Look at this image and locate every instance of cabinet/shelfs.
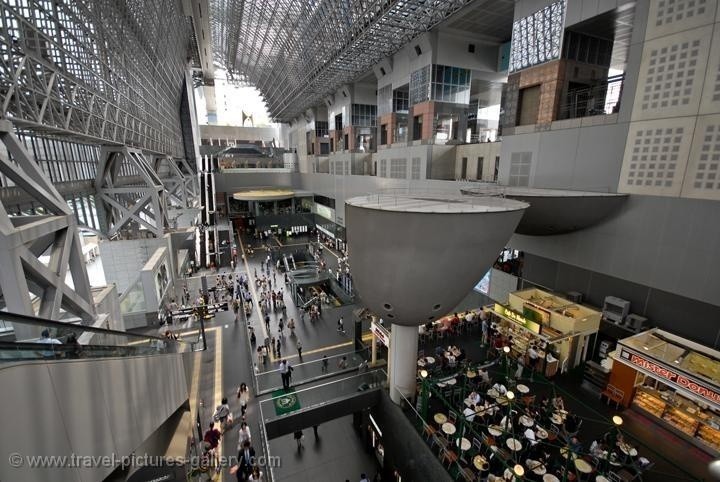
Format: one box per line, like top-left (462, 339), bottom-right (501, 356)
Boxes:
top-left (630, 384), bottom-right (720, 459)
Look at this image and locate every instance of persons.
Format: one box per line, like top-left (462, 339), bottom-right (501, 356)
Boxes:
top-left (156, 221), bottom-right (349, 482)
top-left (217, 206), bottom-right (224, 217)
top-left (417, 307), bottom-right (650, 482)
top-left (64, 331), bottom-right (82, 359)
top-left (359, 467), bottom-right (402, 482)
top-left (35, 329), bottom-right (63, 360)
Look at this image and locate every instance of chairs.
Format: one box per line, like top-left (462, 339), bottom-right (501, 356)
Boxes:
top-left (415, 315), bottom-right (637, 481)
